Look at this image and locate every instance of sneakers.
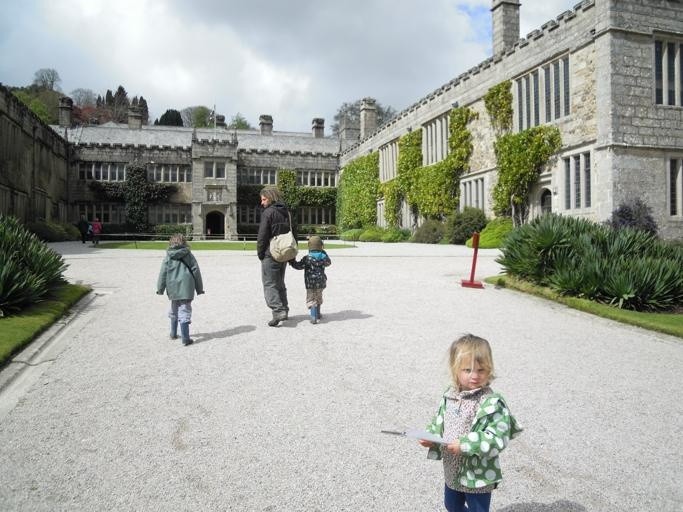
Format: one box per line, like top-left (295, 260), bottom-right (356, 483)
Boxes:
top-left (268, 311), bottom-right (288, 326)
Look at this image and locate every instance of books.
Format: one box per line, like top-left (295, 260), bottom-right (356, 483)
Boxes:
top-left (414, 430), bottom-right (454, 445)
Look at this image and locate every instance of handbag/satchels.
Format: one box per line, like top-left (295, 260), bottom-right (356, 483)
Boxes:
top-left (269, 231), bottom-right (298, 262)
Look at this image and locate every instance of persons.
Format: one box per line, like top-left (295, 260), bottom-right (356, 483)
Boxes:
top-left (155, 234), bottom-right (205, 346)
top-left (91, 217), bottom-right (103, 245)
top-left (256, 185), bottom-right (299, 327)
top-left (77, 215), bottom-right (88, 244)
top-left (288, 235), bottom-right (332, 324)
top-left (414, 333), bottom-right (524, 512)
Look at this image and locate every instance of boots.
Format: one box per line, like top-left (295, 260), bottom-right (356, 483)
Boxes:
top-left (310, 304), bottom-right (320, 324)
top-left (180, 323), bottom-right (193, 345)
top-left (170, 319), bottom-right (177, 338)
